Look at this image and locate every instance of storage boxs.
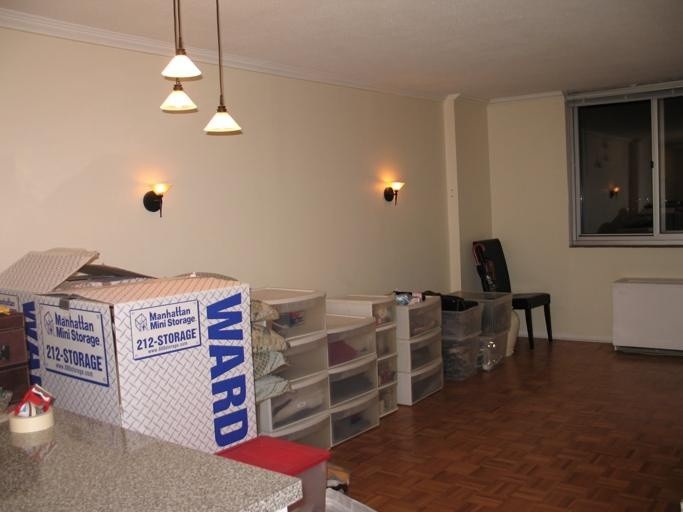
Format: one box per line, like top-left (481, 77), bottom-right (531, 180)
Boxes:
top-left (450, 291), bottom-right (512, 333)
top-left (326, 316), bottom-right (377, 369)
top-left (247, 332), bottom-right (329, 384)
top-left (397, 359), bottom-right (443, 406)
top-left (478, 337), bottom-right (508, 372)
top-left (374, 353), bottom-right (398, 387)
top-left (441, 298), bottom-right (483, 337)
top-left (396, 328), bottom-right (442, 372)
top-left (319, 295), bottom-right (396, 327)
top-left (257, 372), bottom-right (330, 432)
top-left (1, 249), bottom-right (161, 387)
top-left (34, 271), bottom-right (257, 456)
top-left (246, 286), bottom-right (327, 341)
top-left (377, 381), bottom-right (399, 418)
top-left (375, 324), bottom-right (397, 357)
top-left (216, 435), bottom-right (334, 511)
top-left (331, 390), bottom-right (380, 448)
top-left (443, 333), bottom-right (482, 381)
top-left (273, 412), bottom-right (332, 453)
top-left (324, 355), bottom-right (378, 408)
top-left (396, 295), bottom-right (442, 339)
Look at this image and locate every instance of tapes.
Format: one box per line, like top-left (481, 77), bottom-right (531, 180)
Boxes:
top-left (8, 407), bottom-right (56, 434)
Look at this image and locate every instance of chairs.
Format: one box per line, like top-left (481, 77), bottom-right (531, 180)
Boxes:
top-left (471, 238), bottom-right (553, 350)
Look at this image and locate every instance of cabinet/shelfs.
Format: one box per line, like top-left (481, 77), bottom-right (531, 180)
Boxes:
top-left (0, 312), bottom-right (31, 407)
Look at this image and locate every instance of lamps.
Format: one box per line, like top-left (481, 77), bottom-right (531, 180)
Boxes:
top-left (384, 181), bottom-right (404, 207)
top-left (160, 1), bottom-right (197, 111)
top-left (204, 0), bottom-right (241, 132)
top-left (143, 182), bottom-right (172, 218)
top-left (161, 0), bottom-right (202, 79)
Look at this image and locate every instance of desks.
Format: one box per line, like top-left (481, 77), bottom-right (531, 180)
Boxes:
top-left (0, 408), bottom-right (302, 511)
